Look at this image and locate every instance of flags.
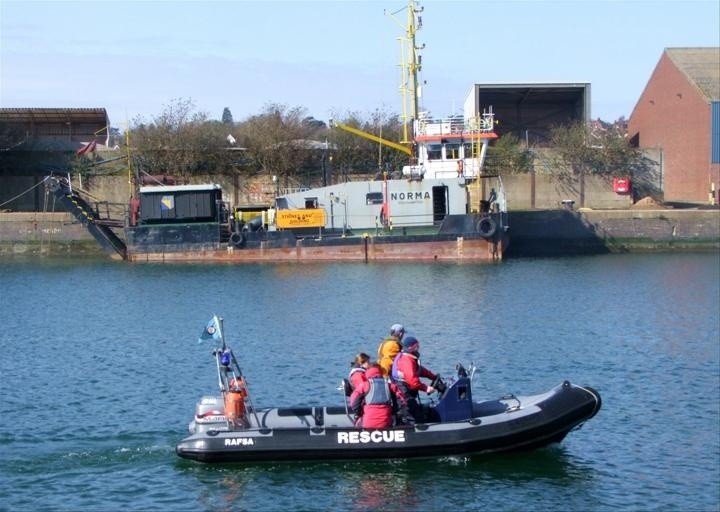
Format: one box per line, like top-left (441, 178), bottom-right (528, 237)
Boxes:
top-left (77, 140), bottom-right (96, 159)
top-left (195, 317), bottom-right (221, 345)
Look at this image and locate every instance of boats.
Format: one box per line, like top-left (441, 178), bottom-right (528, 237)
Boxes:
top-left (43, 0), bottom-right (509, 261)
top-left (175, 313), bottom-right (602, 463)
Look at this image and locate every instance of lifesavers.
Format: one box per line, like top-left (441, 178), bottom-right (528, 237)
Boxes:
top-left (225, 378), bottom-right (246, 426)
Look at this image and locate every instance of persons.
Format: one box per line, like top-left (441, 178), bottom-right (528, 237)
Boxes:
top-left (391, 336), bottom-right (438, 426)
top-left (349, 354), bottom-right (372, 412)
top-left (348, 363), bottom-right (407, 431)
top-left (375, 323), bottom-right (407, 425)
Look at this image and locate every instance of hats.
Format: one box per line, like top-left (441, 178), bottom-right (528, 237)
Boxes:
top-left (403, 337), bottom-right (418, 347)
top-left (390, 324), bottom-right (404, 336)
top-left (365, 367), bottom-right (382, 378)
